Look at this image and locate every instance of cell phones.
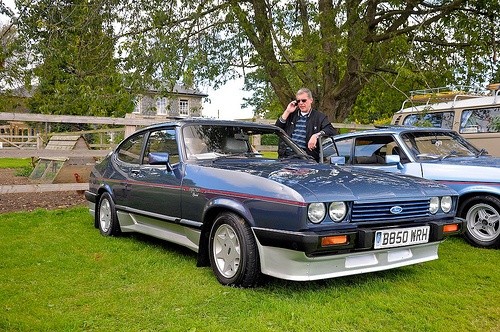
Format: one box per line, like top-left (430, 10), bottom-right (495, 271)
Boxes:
top-left (295, 102), bottom-right (298, 106)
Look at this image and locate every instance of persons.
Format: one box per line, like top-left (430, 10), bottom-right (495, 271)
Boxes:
top-left (275, 88), bottom-right (336, 163)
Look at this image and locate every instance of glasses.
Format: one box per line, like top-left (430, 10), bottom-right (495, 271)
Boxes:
top-left (297, 97), bottom-right (311, 103)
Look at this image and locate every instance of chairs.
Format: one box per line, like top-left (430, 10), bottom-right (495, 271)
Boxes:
top-left (357, 155), bottom-right (384, 164)
top-left (222, 138), bottom-right (248, 156)
top-left (392, 145), bottom-right (411, 164)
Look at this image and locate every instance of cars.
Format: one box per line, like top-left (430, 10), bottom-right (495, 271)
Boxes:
top-left (324, 124), bottom-right (500, 249)
top-left (83, 118), bottom-right (468, 289)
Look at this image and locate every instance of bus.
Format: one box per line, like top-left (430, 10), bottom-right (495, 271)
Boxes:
top-left (390, 85), bottom-right (500, 159)
